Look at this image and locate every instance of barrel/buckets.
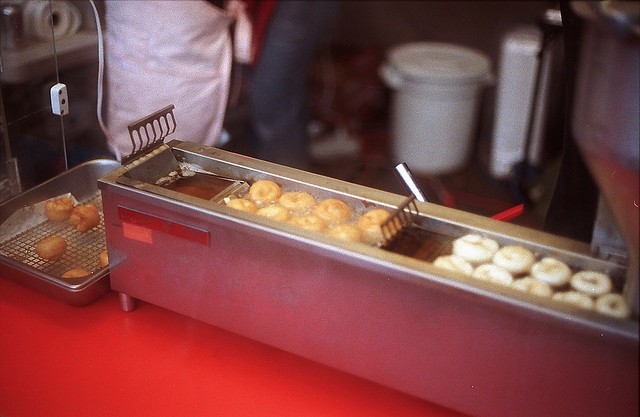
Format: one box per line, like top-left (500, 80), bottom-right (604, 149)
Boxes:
top-left (383, 41), bottom-right (496, 172)
